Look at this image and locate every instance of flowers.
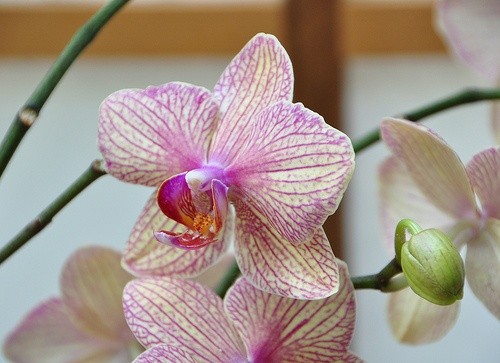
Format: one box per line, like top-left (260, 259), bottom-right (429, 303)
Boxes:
top-left (0, 0), bottom-right (499, 361)
